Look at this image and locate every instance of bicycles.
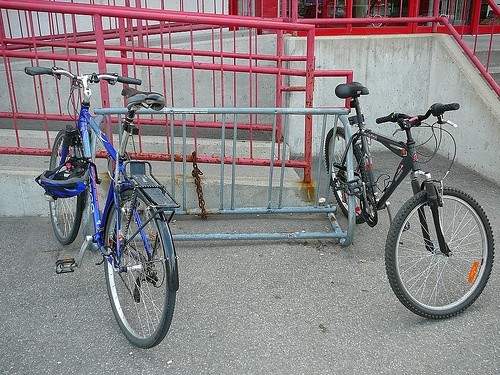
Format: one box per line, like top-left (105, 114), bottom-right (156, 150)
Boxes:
top-left (24, 66), bottom-right (181, 350)
top-left (324, 81), bottom-right (496, 320)
